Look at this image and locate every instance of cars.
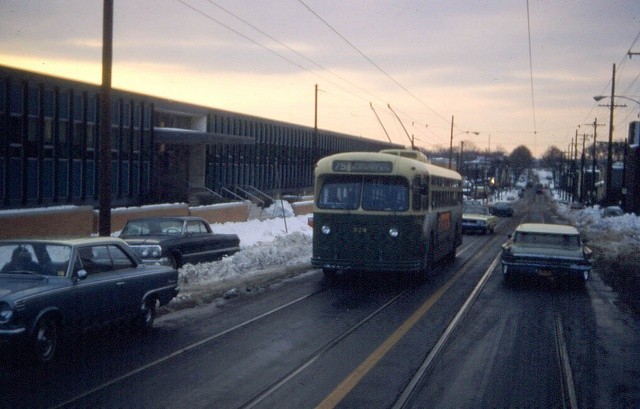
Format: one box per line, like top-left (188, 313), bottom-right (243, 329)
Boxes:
top-left (0, 236), bottom-right (178, 366)
top-left (459, 206), bottom-right (500, 234)
top-left (63, 236), bottom-right (168, 268)
top-left (501, 222), bottom-right (592, 285)
top-left (118, 216), bottom-right (241, 266)
top-left (491, 202), bottom-right (514, 217)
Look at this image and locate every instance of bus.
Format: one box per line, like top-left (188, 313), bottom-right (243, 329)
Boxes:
top-left (310, 149), bottom-right (462, 283)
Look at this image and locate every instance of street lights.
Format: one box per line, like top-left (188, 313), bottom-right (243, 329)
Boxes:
top-left (593, 93), bottom-right (640, 107)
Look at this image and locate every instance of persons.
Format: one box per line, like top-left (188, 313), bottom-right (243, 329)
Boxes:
top-left (327, 187), bottom-right (341, 203)
top-left (0, 247), bottom-right (42, 274)
